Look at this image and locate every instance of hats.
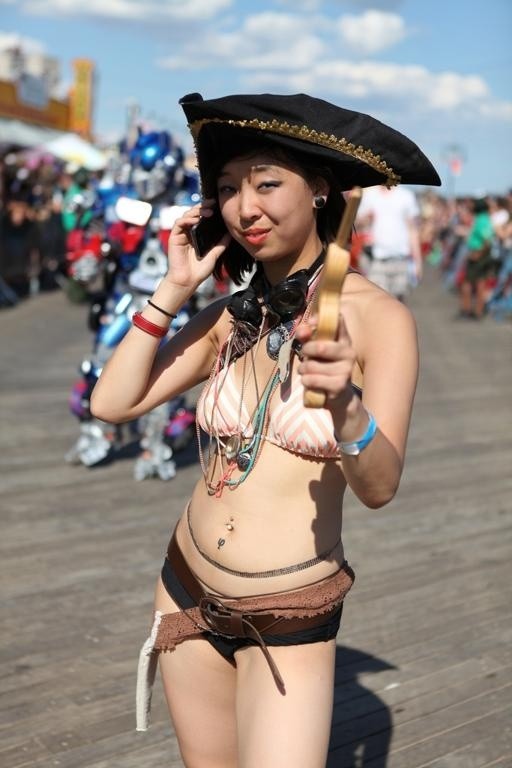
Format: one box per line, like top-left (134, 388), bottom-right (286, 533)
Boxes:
top-left (178, 93), bottom-right (441, 199)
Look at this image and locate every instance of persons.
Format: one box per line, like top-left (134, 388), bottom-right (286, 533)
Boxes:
top-left (84, 145), bottom-right (424, 768)
top-left (1, 146), bottom-right (96, 306)
top-left (61, 126), bottom-right (206, 482)
top-left (352, 181), bottom-right (511, 320)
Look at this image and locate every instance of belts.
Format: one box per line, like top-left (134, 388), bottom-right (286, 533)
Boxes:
top-left (168, 519), bottom-right (342, 696)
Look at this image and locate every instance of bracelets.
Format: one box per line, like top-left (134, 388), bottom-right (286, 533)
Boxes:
top-left (128, 310), bottom-right (170, 340)
top-left (336, 412), bottom-right (380, 457)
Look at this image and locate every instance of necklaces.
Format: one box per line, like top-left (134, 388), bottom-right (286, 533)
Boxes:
top-left (192, 262), bottom-right (327, 500)
